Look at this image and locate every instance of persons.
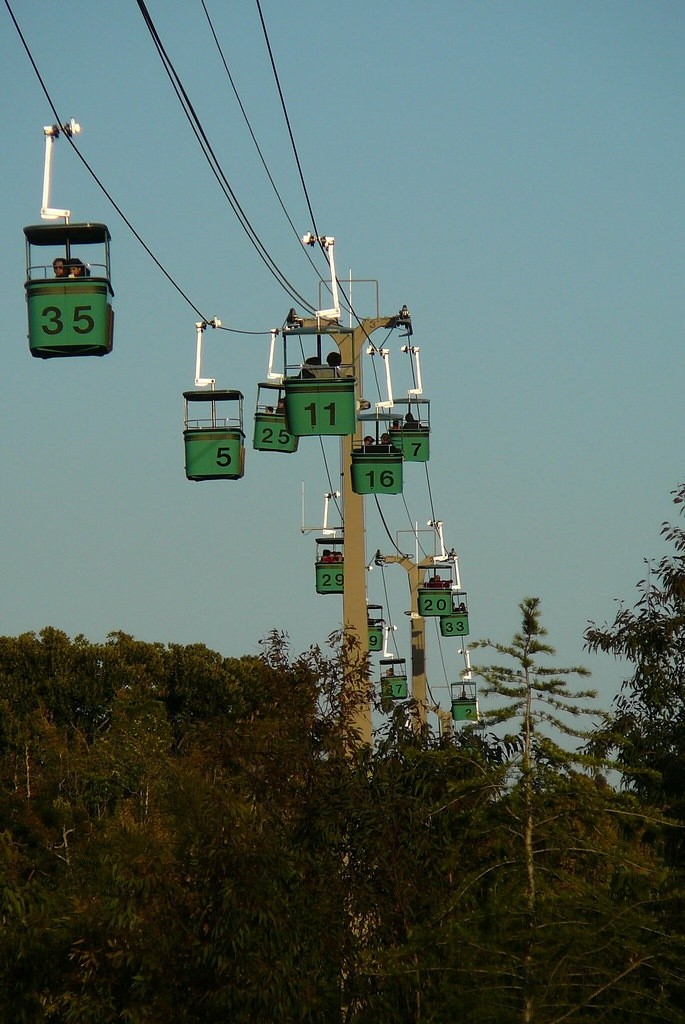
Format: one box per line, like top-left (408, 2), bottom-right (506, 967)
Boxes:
top-left (385, 668), bottom-right (396, 677)
top-left (53, 257), bottom-right (90, 278)
top-left (402, 412), bottom-right (423, 430)
top-left (360, 433), bottom-right (401, 453)
top-left (458, 692), bottom-right (469, 700)
top-left (266, 398), bottom-right (286, 414)
top-left (453, 602), bottom-right (466, 611)
top-left (429, 575), bottom-right (442, 589)
top-left (321, 549), bottom-right (344, 562)
top-left (367, 613), bottom-right (387, 627)
top-left (390, 420), bottom-right (401, 430)
top-left (297, 352), bottom-right (342, 378)
